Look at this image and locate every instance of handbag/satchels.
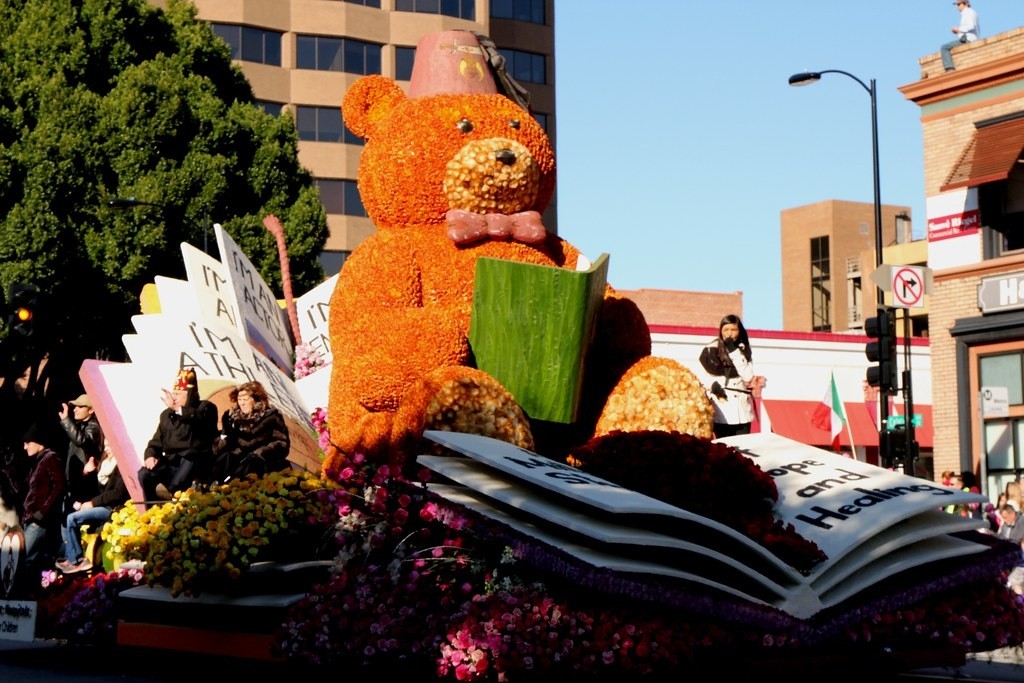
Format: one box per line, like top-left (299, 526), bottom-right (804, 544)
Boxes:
top-left (700, 347), bottom-right (738, 378)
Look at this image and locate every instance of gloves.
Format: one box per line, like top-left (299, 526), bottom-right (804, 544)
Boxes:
top-left (712, 381), bottom-right (727, 399)
top-left (724, 337), bottom-right (737, 352)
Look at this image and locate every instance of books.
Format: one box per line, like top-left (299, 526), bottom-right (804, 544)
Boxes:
top-left (462, 252), bottom-right (610, 426)
top-left (80, 217), bottom-right (347, 505)
top-left (417, 431), bottom-right (991, 619)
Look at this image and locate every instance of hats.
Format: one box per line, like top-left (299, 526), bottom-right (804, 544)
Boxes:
top-left (68, 394), bottom-right (91, 408)
top-left (172, 367), bottom-right (199, 392)
top-left (21, 423), bottom-right (48, 446)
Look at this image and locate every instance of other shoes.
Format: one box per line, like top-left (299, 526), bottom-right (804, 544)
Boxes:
top-left (62, 556), bottom-right (92, 573)
top-left (55, 559), bottom-right (71, 570)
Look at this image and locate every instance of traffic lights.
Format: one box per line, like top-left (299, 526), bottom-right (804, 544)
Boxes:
top-left (7, 280), bottom-right (37, 350)
top-left (864, 312), bottom-right (902, 396)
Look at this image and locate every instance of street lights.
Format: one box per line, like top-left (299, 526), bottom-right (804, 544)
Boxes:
top-left (787, 69), bottom-right (890, 468)
top-left (102, 195), bottom-right (210, 257)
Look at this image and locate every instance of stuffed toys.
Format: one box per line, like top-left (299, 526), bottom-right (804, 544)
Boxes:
top-left (330, 61), bottom-right (713, 477)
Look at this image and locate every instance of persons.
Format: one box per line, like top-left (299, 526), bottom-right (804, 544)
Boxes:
top-left (57, 394), bottom-right (104, 543)
top-left (210, 381), bottom-right (291, 483)
top-left (937, 470), bottom-right (1024, 549)
top-left (17, 430), bottom-right (64, 583)
top-left (53, 434), bottom-right (132, 575)
top-left (137, 365), bottom-right (219, 509)
top-left (694, 315), bottom-right (755, 440)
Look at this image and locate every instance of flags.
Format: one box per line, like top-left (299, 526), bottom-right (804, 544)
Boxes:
top-left (864, 378), bottom-right (899, 433)
top-left (810, 369), bottom-right (849, 455)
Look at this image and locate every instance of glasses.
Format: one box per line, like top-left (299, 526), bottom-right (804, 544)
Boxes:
top-left (949, 482), bottom-right (962, 486)
